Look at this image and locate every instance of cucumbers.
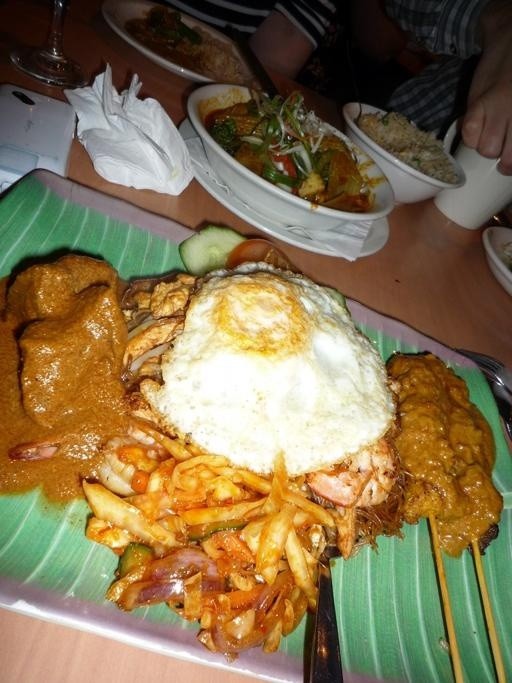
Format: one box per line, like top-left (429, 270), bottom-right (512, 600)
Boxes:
top-left (179, 224), bottom-right (246, 274)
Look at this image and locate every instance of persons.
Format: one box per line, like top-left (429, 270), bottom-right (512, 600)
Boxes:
top-left (153, 0), bottom-right (340, 103)
top-left (379, 0), bottom-right (512, 178)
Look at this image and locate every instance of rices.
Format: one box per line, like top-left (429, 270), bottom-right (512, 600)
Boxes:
top-left (357, 111), bottom-right (456, 184)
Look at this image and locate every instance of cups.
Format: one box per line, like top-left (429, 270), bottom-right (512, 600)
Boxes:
top-left (430, 111), bottom-right (512, 233)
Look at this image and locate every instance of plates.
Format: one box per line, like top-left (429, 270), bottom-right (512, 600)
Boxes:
top-left (0, 164), bottom-right (512, 683)
top-left (177, 116), bottom-right (390, 259)
top-left (101, 0), bottom-right (258, 86)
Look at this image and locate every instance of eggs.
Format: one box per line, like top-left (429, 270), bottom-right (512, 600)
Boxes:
top-left (139, 262), bottom-right (395, 479)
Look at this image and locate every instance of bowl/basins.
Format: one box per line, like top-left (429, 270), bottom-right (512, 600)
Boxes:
top-left (340, 95), bottom-right (467, 205)
top-left (480, 225), bottom-right (512, 297)
top-left (184, 81), bottom-right (397, 231)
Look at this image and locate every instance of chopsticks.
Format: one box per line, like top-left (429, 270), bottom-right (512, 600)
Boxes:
top-left (224, 19), bottom-right (281, 102)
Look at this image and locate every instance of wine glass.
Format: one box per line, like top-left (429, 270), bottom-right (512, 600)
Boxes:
top-left (8, 1), bottom-right (95, 90)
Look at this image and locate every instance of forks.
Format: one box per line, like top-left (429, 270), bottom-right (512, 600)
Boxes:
top-left (451, 344), bottom-right (511, 397)
